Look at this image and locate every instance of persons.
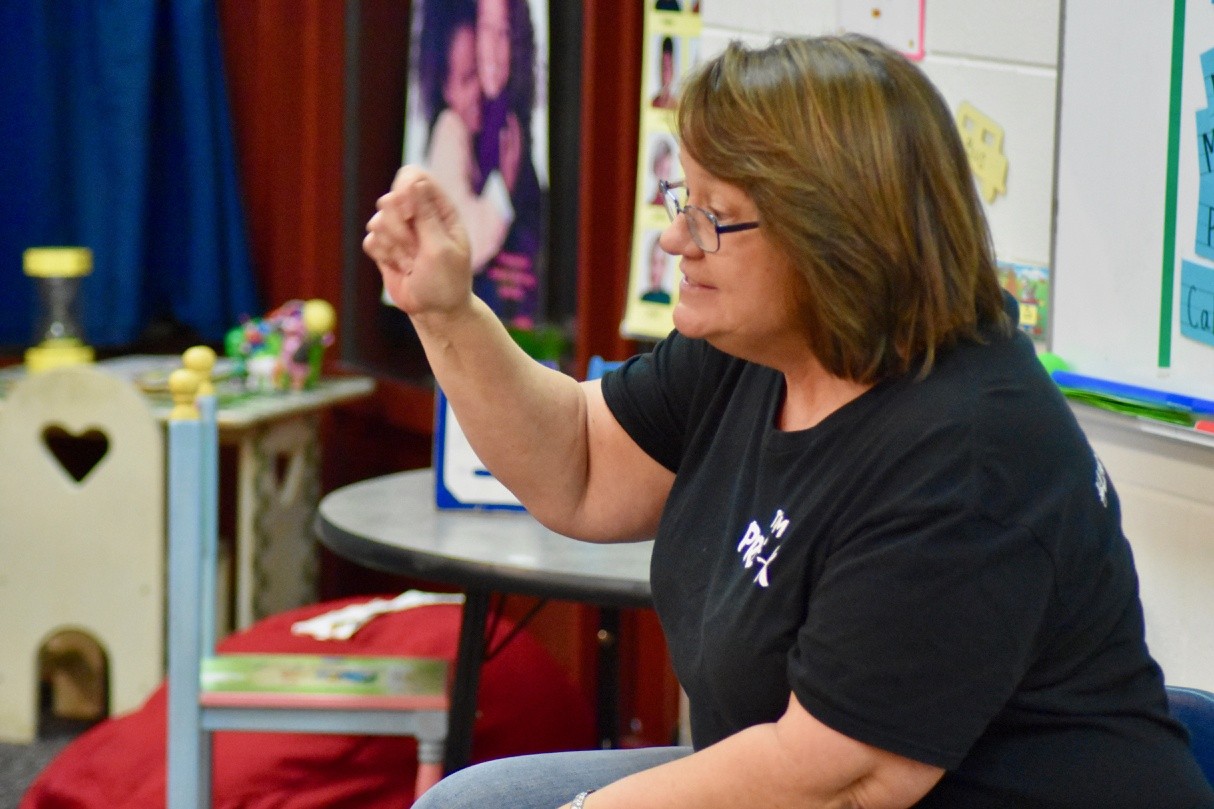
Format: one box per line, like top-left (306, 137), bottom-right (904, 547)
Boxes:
top-left (362, 31), bottom-right (1214, 809)
top-left (419, 0), bottom-right (543, 317)
top-left (650, 141), bottom-right (673, 205)
top-left (641, 237), bottom-right (671, 304)
top-left (653, 37), bottom-right (677, 109)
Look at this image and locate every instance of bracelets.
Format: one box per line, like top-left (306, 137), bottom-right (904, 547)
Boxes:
top-left (570, 789), bottom-right (594, 809)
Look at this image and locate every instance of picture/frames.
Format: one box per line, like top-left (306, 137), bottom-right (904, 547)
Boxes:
top-left (343, 0), bottom-right (584, 390)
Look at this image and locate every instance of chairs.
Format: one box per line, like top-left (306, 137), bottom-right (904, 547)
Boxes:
top-left (166, 347), bottom-right (451, 809)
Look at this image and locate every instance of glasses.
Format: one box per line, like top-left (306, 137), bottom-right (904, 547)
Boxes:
top-left (658, 179), bottom-right (759, 253)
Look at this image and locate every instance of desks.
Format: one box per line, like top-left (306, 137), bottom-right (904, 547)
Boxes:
top-left (306, 465), bottom-right (653, 774)
